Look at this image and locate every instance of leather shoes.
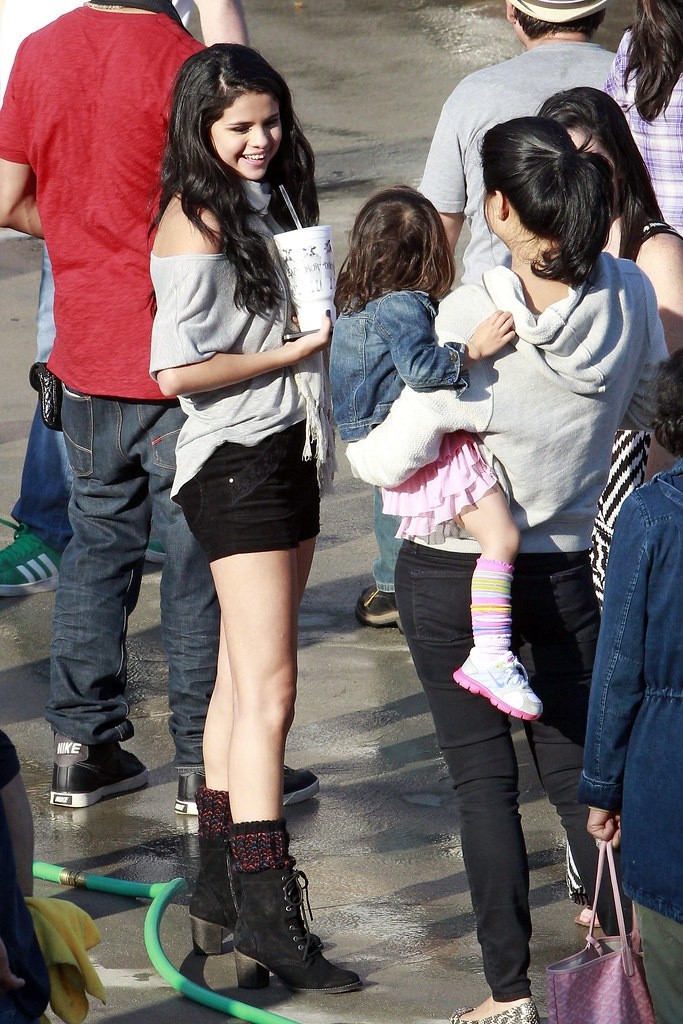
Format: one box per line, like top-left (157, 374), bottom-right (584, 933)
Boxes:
top-left (450, 1000), bottom-right (540, 1024)
top-left (357, 585), bottom-right (403, 633)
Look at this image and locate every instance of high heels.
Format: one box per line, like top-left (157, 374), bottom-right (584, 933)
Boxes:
top-left (233, 865), bottom-right (363, 994)
top-left (190, 836), bottom-right (239, 958)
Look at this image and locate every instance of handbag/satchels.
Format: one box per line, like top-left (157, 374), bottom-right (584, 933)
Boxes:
top-left (546, 840), bottom-right (656, 1024)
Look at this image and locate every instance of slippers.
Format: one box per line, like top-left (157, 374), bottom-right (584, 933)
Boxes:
top-left (574, 905), bottom-right (601, 927)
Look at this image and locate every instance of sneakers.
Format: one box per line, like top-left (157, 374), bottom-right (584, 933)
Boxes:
top-left (174, 765), bottom-right (321, 816)
top-left (48, 733), bottom-right (150, 808)
top-left (453, 651), bottom-right (543, 720)
top-left (0, 519), bottom-right (63, 595)
top-left (145, 538), bottom-right (169, 564)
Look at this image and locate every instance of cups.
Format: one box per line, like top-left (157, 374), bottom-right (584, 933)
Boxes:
top-left (270, 225), bottom-right (336, 333)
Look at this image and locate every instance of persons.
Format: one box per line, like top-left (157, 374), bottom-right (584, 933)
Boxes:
top-left (1, 804), bottom-right (107, 1024)
top-left (326, 186), bottom-right (542, 721)
top-left (577, 346), bottom-right (683, 1024)
top-left (607, 0), bottom-right (683, 236)
top-left (1, 730), bottom-right (32, 896)
top-left (144, 44), bottom-right (360, 994)
top-left (391, 118), bottom-right (667, 1024)
top-left (0, 0), bottom-right (194, 601)
top-left (415, 0), bottom-right (615, 285)
top-left (536, 87), bottom-right (682, 355)
top-left (0, 0), bottom-right (323, 816)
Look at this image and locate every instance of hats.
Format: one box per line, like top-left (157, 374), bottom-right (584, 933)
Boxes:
top-left (509, 0), bottom-right (609, 23)
